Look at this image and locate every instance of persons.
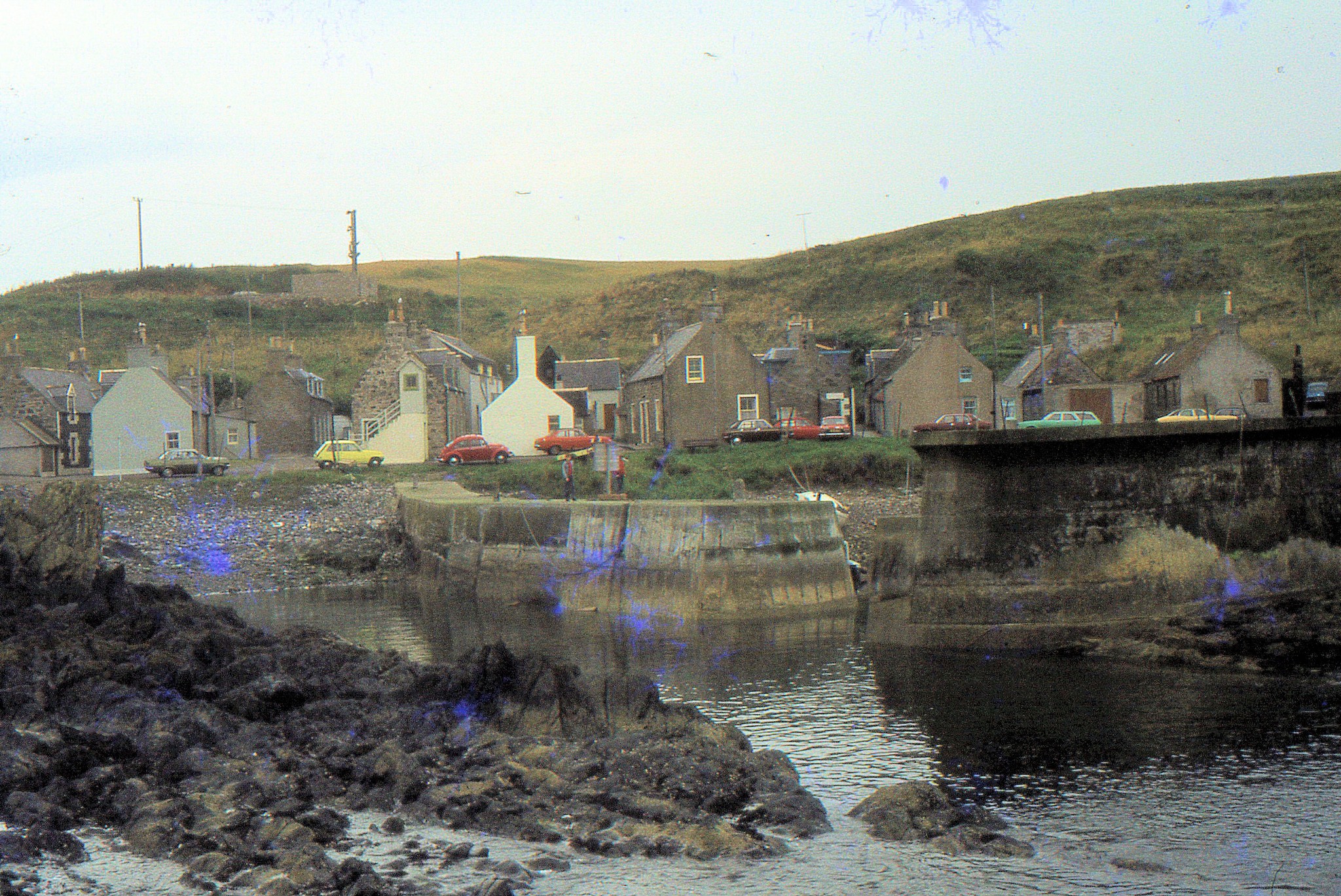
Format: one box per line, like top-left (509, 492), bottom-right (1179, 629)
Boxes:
top-left (614, 455), bottom-right (626, 496)
top-left (562, 454), bottom-right (577, 503)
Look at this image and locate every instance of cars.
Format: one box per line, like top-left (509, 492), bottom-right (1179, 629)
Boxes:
top-left (911, 412), bottom-right (993, 432)
top-left (435, 434), bottom-right (513, 466)
top-left (721, 417), bottom-right (782, 444)
top-left (1016, 411), bottom-right (1104, 429)
top-left (144, 448), bottom-right (233, 482)
top-left (770, 417), bottom-right (822, 441)
top-left (534, 428), bottom-right (615, 456)
top-left (1152, 407), bottom-right (1240, 423)
top-left (1303, 380), bottom-right (1332, 409)
top-left (313, 439), bottom-right (387, 469)
top-left (816, 416), bottom-right (852, 440)
top-left (1216, 407), bottom-right (1246, 418)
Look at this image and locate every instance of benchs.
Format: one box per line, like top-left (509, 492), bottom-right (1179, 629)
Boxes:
top-left (682, 438), bottom-right (719, 453)
